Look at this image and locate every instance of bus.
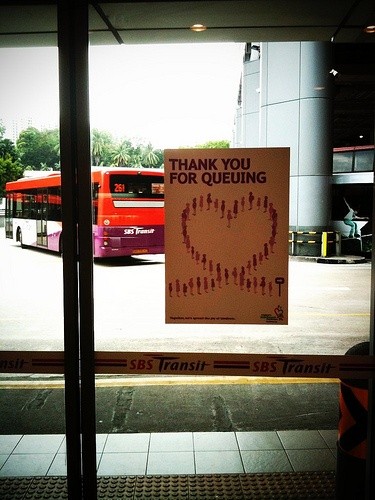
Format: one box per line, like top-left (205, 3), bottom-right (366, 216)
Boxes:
top-left (5, 163), bottom-right (166, 262)
